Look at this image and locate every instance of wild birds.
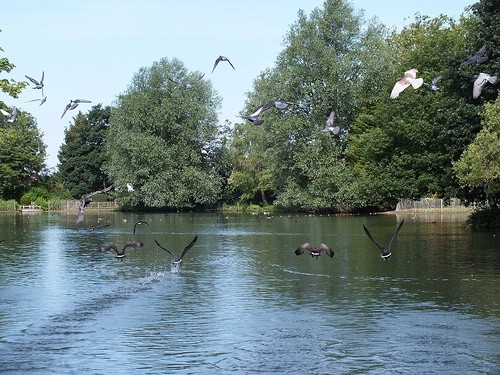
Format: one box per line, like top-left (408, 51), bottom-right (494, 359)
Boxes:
top-left (76, 185), bottom-right (112, 224)
top-left (36, 131), bottom-right (45, 138)
top-left (265, 99), bottom-right (295, 113)
top-left (154, 235), bottom-right (198, 266)
top-left (211, 55), bottom-right (235, 72)
top-left (297, 104), bottom-right (309, 110)
top-left (127, 183), bottom-right (134, 193)
top-left (25, 71), bottom-right (45, 90)
top-left (99, 241), bottom-right (143, 262)
top-left (28, 145), bottom-right (41, 156)
top-left (167, 73), bottom-right (205, 89)
top-left (133, 221), bottom-right (153, 235)
top-left (60, 99), bottom-right (92, 120)
top-left (294, 242), bottom-right (337, 258)
top-left (0, 106), bottom-right (18, 123)
top-left (237, 103), bottom-right (267, 125)
top-left (390, 45), bottom-right (497, 99)
top-left (364, 220), bottom-right (405, 261)
top-left (26, 88), bottom-right (47, 107)
top-left (322, 112), bottom-right (345, 136)
